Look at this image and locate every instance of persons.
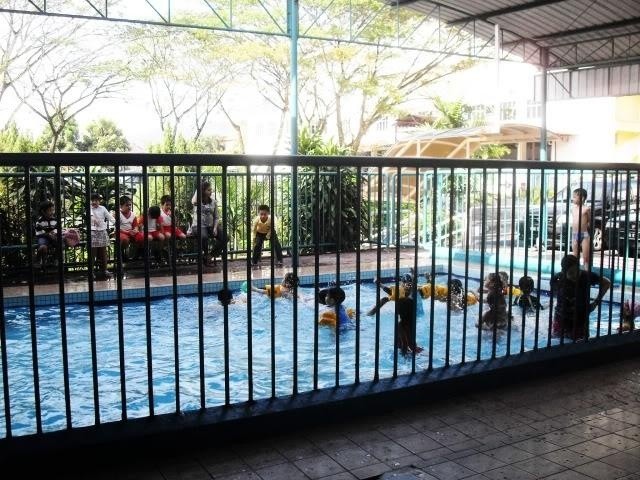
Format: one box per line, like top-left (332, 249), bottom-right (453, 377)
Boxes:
top-left (216, 289), bottom-right (237, 303)
top-left (319, 287), bottom-right (351, 326)
top-left (34, 201), bottom-right (57, 266)
top-left (251, 272), bottom-right (300, 299)
top-left (251, 204), bottom-right (283, 266)
top-left (617, 299), bottom-right (640, 330)
top-left (396, 282), bottom-right (423, 353)
top-left (137, 205), bottom-right (166, 249)
top-left (550, 255), bottom-right (611, 340)
top-left (114, 196), bottom-right (144, 250)
top-left (160, 194), bottom-right (187, 241)
top-left (191, 181), bottom-right (230, 266)
top-left (90, 192), bottom-right (116, 278)
top-left (367, 272), bottom-right (542, 329)
top-left (571, 188), bottom-right (591, 271)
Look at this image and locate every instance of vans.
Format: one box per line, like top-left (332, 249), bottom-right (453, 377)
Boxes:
top-left (515, 180), bottom-right (636, 250)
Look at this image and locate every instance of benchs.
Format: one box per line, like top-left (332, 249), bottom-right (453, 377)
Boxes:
top-left (0, 239), bottom-right (69, 285)
top-left (125, 232), bottom-right (217, 272)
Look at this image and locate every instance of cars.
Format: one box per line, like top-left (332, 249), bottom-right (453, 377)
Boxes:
top-left (605, 207), bottom-right (639, 259)
